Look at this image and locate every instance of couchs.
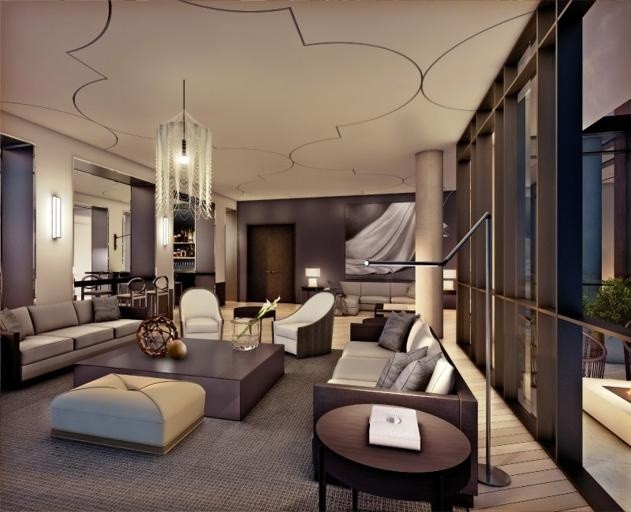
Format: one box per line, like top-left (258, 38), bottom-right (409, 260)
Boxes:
top-left (314, 322), bottom-right (479, 499)
top-left (272, 291), bottom-right (335, 360)
top-left (324, 278), bottom-right (415, 315)
top-left (180, 286), bottom-right (224, 341)
top-left (0, 302), bottom-right (146, 390)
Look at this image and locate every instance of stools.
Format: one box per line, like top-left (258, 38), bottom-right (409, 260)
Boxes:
top-left (49, 373), bottom-right (207, 454)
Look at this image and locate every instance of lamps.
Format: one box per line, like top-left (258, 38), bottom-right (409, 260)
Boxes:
top-left (52, 195), bottom-right (61, 240)
top-left (304, 267), bottom-right (321, 288)
top-left (162, 217), bottom-right (170, 248)
top-left (156, 78), bottom-right (214, 221)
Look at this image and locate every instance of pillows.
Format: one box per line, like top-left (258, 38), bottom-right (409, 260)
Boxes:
top-left (328, 280), bottom-right (345, 295)
top-left (27, 302), bottom-right (79, 335)
top-left (376, 347), bottom-right (428, 391)
top-left (93, 294), bottom-right (120, 319)
top-left (0, 307), bottom-right (23, 342)
top-left (73, 298), bottom-right (93, 324)
top-left (10, 306), bottom-right (34, 336)
top-left (390, 350), bottom-right (443, 392)
top-left (377, 312), bottom-right (417, 352)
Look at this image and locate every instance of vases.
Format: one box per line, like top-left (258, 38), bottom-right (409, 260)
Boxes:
top-left (231, 320), bottom-right (260, 351)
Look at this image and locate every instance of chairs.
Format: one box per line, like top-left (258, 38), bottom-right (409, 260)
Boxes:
top-left (118, 277), bottom-right (148, 314)
top-left (149, 274), bottom-right (170, 317)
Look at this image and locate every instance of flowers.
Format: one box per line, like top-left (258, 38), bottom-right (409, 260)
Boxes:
top-left (237, 296), bottom-right (280, 339)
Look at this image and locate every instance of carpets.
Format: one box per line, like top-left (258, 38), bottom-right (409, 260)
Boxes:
top-left (1, 349), bottom-right (470, 512)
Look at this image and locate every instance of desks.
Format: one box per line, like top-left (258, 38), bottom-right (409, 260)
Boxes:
top-left (74, 276), bottom-right (156, 305)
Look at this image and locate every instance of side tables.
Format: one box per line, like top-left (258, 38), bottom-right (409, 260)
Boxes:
top-left (233, 306), bottom-right (276, 345)
top-left (314, 404), bottom-right (471, 512)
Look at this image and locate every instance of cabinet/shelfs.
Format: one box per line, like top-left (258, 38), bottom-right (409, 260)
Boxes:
top-left (174, 204), bottom-right (214, 273)
